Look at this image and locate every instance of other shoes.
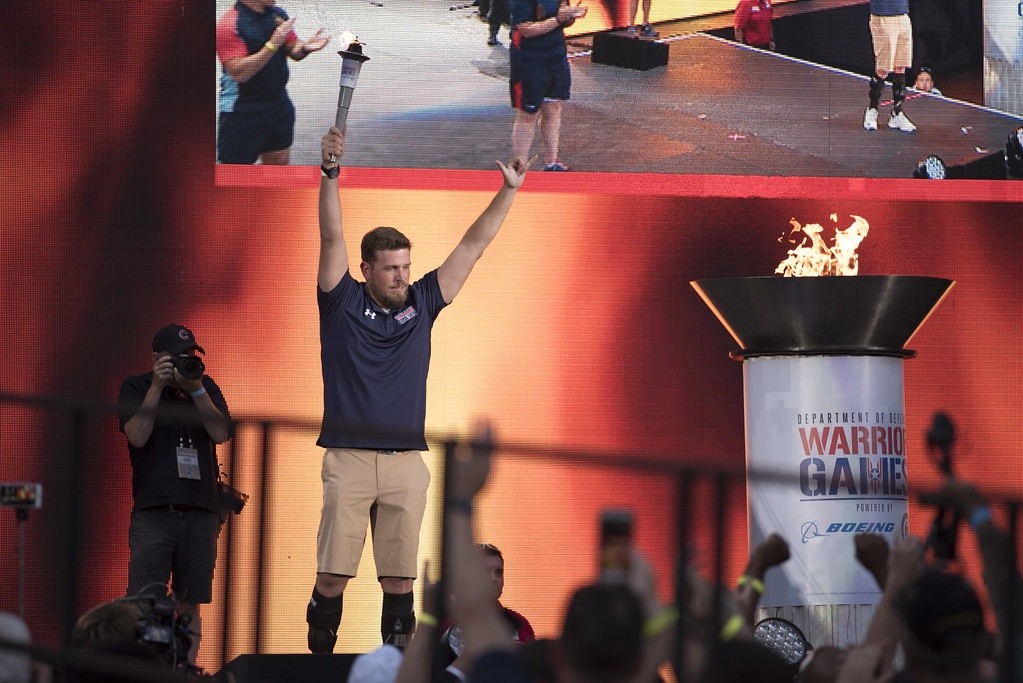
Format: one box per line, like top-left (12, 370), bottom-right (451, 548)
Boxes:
top-left (641, 25), bottom-right (658, 37)
top-left (487, 37), bottom-right (496, 45)
top-left (544, 163), bottom-right (571, 172)
top-left (628, 28), bottom-right (639, 39)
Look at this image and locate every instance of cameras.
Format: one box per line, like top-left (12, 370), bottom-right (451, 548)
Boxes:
top-left (167, 352), bottom-right (205, 380)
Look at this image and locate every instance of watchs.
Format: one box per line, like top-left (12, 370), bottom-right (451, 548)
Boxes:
top-left (321, 164), bottom-right (340, 179)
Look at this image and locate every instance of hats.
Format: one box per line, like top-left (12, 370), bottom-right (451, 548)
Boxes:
top-left (153, 324), bottom-right (206, 356)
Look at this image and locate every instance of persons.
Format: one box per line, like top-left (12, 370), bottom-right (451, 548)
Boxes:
top-left (304, 125), bottom-right (537, 653)
top-left (476, 0), bottom-right (588, 172)
top-left (733, 0), bottom-right (776, 52)
top-left (116, 322), bottom-right (233, 664)
top-left (628, 0), bottom-right (658, 39)
top-left (863, 0), bottom-right (917, 131)
top-left (216, 0), bottom-right (332, 166)
top-left (912, 67), bottom-right (942, 95)
top-left (0, 414), bottom-right (1023, 683)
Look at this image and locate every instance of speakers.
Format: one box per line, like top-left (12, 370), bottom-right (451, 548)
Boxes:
top-left (210, 654), bottom-right (364, 683)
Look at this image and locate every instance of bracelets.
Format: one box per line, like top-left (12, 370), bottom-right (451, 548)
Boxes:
top-left (445, 498), bottom-right (472, 513)
top-left (419, 612), bottom-right (439, 626)
top-left (190, 386), bottom-right (206, 397)
top-left (969, 507), bottom-right (989, 525)
top-left (556, 16), bottom-right (563, 26)
top-left (719, 613), bottom-right (745, 640)
top-left (641, 605), bottom-right (678, 635)
top-left (265, 41), bottom-right (278, 53)
top-left (738, 574), bottom-right (765, 594)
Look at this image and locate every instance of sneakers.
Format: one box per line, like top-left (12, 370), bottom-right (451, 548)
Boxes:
top-left (888, 111), bottom-right (915, 132)
top-left (864, 106), bottom-right (878, 130)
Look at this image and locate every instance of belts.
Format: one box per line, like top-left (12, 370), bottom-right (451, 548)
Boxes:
top-left (152, 502), bottom-right (202, 512)
